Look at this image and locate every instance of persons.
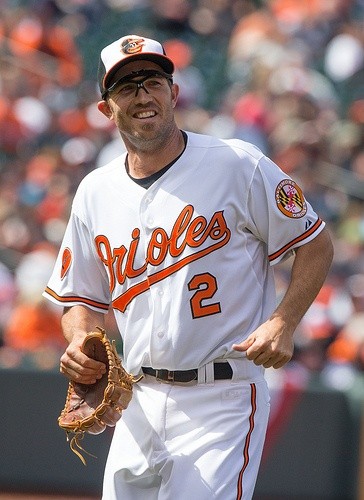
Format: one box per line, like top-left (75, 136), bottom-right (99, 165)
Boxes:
top-left (2, 0), bottom-right (364, 374)
top-left (319, 311), bottom-right (364, 500)
top-left (40, 31), bottom-right (334, 500)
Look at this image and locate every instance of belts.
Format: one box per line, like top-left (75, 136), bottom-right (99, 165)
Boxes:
top-left (138, 360), bottom-right (232, 383)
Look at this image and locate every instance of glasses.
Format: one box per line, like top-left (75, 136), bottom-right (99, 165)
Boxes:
top-left (105, 70), bottom-right (170, 99)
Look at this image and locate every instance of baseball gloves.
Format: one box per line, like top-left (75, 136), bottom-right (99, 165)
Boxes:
top-left (59, 326), bottom-right (145, 465)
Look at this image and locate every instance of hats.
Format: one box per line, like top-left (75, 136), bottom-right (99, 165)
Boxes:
top-left (98, 35), bottom-right (175, 96)
